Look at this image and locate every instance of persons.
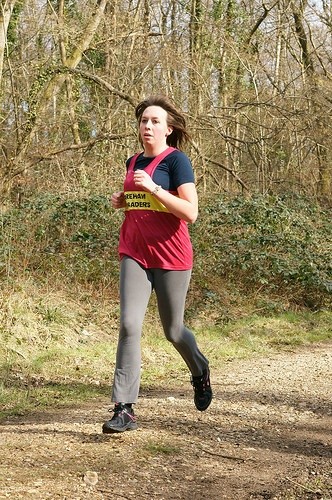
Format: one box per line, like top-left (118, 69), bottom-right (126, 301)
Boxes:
top-left (102, 95), bottom-right (213, 432)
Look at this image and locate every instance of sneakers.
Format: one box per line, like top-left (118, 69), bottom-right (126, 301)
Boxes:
top-left (190, 365), bottom-right (212, 412)
top-left (102, 402), bottom-right (137, 432)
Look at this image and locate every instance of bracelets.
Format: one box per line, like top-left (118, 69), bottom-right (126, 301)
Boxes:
top-left (151, 185), bottom-right (161, 195)
